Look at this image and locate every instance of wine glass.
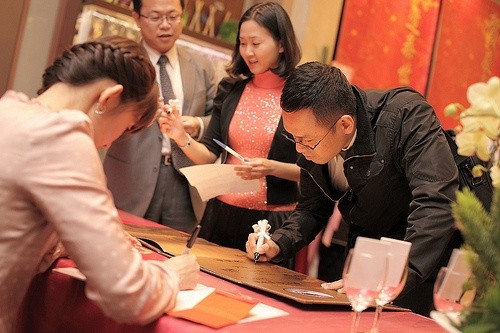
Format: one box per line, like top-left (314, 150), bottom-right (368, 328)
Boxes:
top-left (369, 254), bottom-right (409, 333)
top-left (341, 248), bottom-right (388, 333)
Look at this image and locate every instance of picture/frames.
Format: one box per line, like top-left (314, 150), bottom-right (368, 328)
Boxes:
top-left (71, 0), bottom-right (236, 85)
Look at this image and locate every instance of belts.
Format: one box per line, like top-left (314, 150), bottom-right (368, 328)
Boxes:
top-left (160, 154), bottom-right (173, 167)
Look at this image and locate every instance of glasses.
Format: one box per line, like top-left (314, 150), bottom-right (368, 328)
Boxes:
top-left (280, 112), bottom-right (355, 152)
top-left (138, 12), bottom-right (185, 25)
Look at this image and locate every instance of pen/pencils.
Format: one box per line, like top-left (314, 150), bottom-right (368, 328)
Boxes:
top-left (183, 224), bottom-right (202, 255)
top-left (167, 107), bottom-right (172, 114)
top-left (213, 138), bottom-right (249, 163)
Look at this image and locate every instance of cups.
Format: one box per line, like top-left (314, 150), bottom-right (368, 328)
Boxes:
top-left (434, 266), bottom-right (477, 313)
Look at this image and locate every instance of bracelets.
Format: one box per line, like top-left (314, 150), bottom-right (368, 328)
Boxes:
top-left (179, 133), bottom-right (192, 150)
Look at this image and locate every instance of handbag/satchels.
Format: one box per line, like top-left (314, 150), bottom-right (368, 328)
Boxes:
top-left (440, 129), bottom-right (494, 213)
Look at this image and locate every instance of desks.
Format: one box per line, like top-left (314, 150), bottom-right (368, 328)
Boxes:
top-left (24, 207), bottom-right (448, 333)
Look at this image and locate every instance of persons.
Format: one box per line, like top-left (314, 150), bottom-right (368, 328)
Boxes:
top-left (159, 2), bottom-right (302, 270)
top-left (245, 61), bottom-right (460, 318)
top-left (102, 0), bottom-right (228, 235)
top-left (0, 36), bottom-right (201, 333)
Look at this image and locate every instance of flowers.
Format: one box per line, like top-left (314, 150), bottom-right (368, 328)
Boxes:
top-left (443, 75), bottom-right (500, 333)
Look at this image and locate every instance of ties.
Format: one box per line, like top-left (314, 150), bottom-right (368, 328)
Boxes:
top-left (157, 54), bottom-right (193, 176)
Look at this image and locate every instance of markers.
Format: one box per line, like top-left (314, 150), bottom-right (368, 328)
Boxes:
top-left (253, 219), bottom-right (268, 265)
